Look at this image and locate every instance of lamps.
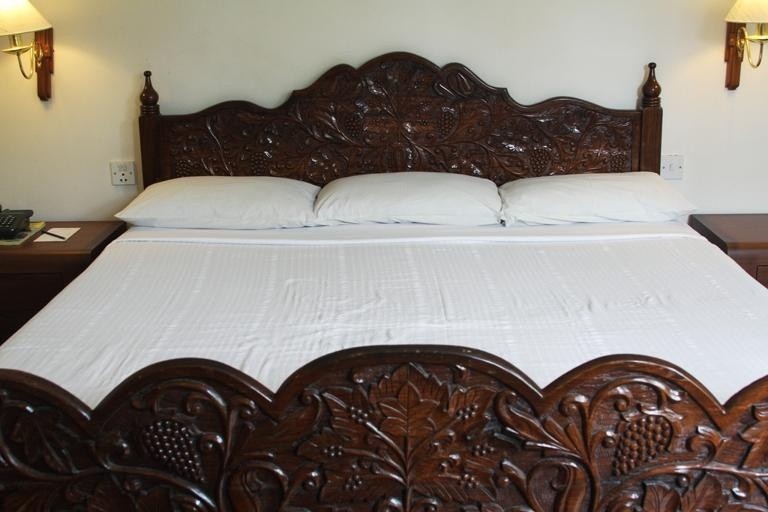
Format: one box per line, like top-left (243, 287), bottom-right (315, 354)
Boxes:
top-left (722, 0), bottom-right (767, 90)
top-left (0, 1), bottom-right (55, 102)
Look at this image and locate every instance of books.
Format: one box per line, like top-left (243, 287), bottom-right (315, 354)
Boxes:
top-left (0, 221), bottom-right (45, 246)
top-left (33, 227), bottom-right (80, 243)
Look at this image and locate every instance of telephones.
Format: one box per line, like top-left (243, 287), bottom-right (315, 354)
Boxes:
top-left (1, 209), bottom-right (33, 238)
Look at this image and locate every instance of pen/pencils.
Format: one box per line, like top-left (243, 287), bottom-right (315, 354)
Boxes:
top-left (42, 230), bottom-right (64, 240)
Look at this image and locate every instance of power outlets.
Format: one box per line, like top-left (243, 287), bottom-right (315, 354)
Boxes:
top-left (110, 160), bottom-right (137, 187)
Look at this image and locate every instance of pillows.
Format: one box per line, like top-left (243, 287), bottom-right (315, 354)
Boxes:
top-left (311, 170), bottom-right (505, 226)
top-left (495, 170), bottom-right (697, 225)
top-left (113, 175), bottom-right (323, 230)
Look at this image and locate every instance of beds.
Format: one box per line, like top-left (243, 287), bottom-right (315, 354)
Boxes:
top-left (0, 52), bottom-right (767, 511)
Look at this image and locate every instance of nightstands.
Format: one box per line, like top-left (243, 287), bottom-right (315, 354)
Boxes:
top-left (687, 213), bottom-right (767, 289)
top-left (1, 220), bottom-right (125, 346)
